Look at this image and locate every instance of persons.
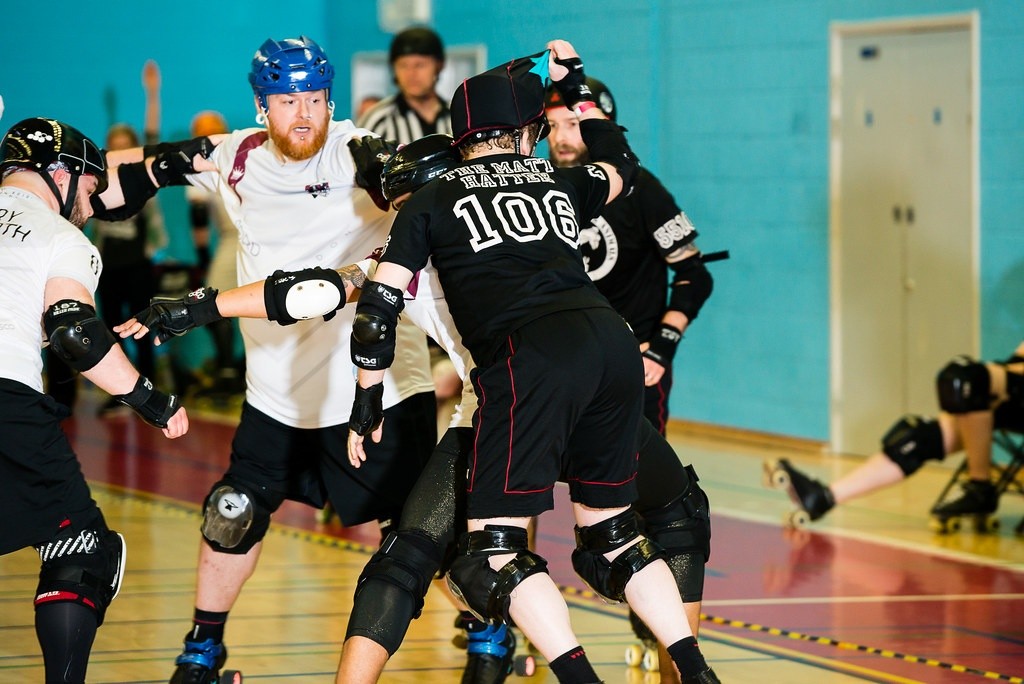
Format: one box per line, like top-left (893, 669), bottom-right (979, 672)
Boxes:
top-left (105, 36), bottom-right (438, 683)
top-left (190, 112), bottom-right (236, 401)
top-left (93, 127), bottom-right (165, 420)
top-left (763, 341), bottom-right (1023, 552)
top-left (355, 25), bottom-right (453, 143)
top-left (1, 116), bottom-right (225, 683)
top-left (112, 37), bottom-right (713, 683)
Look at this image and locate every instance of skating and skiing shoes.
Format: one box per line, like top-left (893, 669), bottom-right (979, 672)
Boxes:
top-left (764, 458), bottom-right (832, 529)
top-left (168, 630), bottom-right (242, 684)
top-left (625, 609), bottom-right (661, 671)
top-left (929, 479), bottom-right (1001, 534)
top-left (455, 615), bottom-right (536, 684)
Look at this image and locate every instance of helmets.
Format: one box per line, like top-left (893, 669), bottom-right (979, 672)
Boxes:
top-left (544, 76), bottom-right (616, 121)
top-left (390, 26), bottom-right (444, 64)
top-left (0, 117), bottom-right (108, 197)
top-left (382, 135), bottom-right (463, 200)
top-left (249, 36), bottom-right (334, 94)
top-left (450, 75), bottom-right (551, 144)
